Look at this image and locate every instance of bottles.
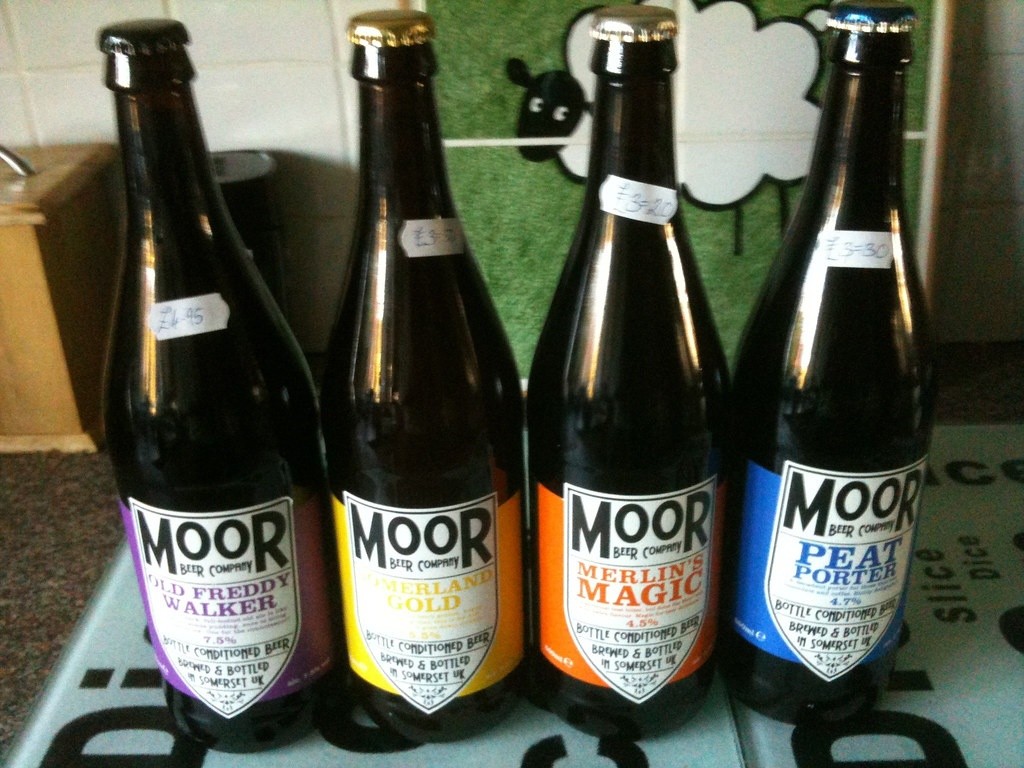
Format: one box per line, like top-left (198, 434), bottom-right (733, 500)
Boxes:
top-left (731, 7), bottom-right (932, 731)
top-left (311, 9), bottom-right (533, 747)
top-left (524, 5), bottom-right (736, 747)
top-left (195, 148), bottom-right (292, 337)
top-left (90, 18), bottom-right (345, 756)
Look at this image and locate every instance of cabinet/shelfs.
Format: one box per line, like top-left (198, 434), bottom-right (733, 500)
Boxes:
top-left (1, 138), bottom-right (130, 456)
